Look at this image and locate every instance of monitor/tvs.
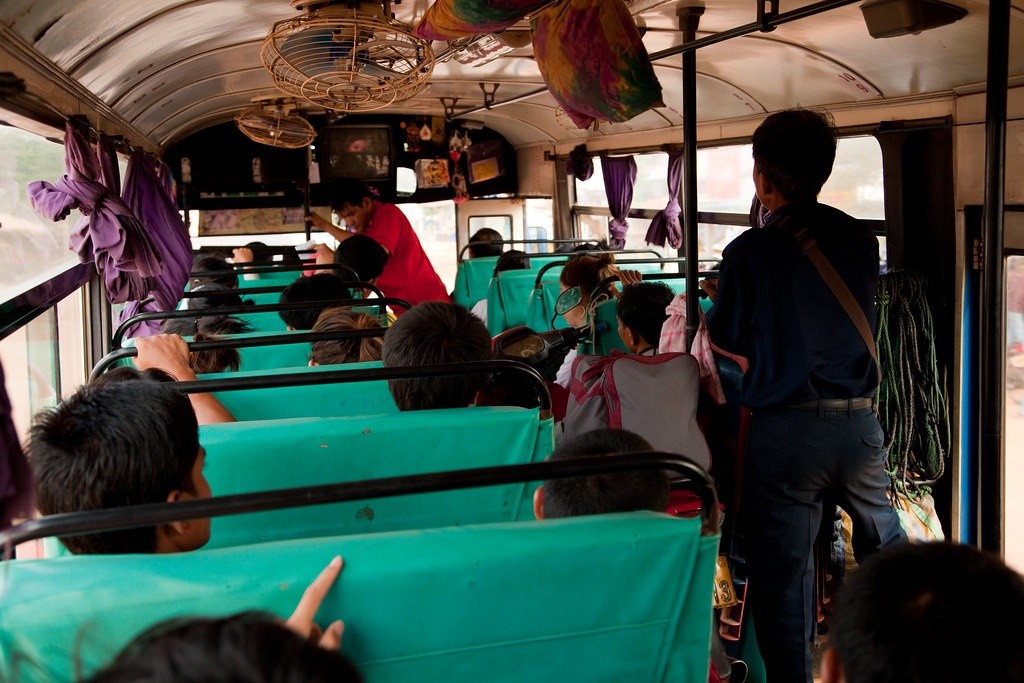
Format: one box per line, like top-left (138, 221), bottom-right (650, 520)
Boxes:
top-left (318, 123), bottom-right (397, 193)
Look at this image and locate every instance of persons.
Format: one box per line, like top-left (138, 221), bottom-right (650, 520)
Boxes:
top-left (569, 239), bottom-right (612, 261)
top-left (448, 228), bottom-right (504, 302)
top-left (609, 270), bottom-right (678, 357)
top-left (29, 234), bottom-right (666, 553)
top-left (471, 250), bottom-right (531, 328)
top-left (697, 107), bottom-right (1024, 683)
top-left (79, 554), bottom-right (368, 683)
top-left (305, 177), bottom-right (453, 318)
top-left (553, 252), bottom-right (620, 387)
top-left (333, 132), bottom-right (388, 180)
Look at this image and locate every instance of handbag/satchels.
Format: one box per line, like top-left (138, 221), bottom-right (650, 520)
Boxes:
top-left (659, 293), bottom-right (750, 406)
top-left (563, 349), bottom-right (712, 474)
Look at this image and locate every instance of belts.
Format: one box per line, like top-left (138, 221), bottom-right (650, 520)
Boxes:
top-left (787, 399), bottom-right (873, 410)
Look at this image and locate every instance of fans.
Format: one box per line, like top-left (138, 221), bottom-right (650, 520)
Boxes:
top-left (234, 93), bottom-right (317, 148)
top-left (258, 0), bottom-right (435, 113)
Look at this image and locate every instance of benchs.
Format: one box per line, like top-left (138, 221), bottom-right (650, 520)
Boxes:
top-left (0, 239), bottom-right (722, 683)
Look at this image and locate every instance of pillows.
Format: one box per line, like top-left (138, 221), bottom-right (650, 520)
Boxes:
top-left (412, 0), bottom-right (551, 41)
top-left (530, 0), bottom-right (667, 129)
